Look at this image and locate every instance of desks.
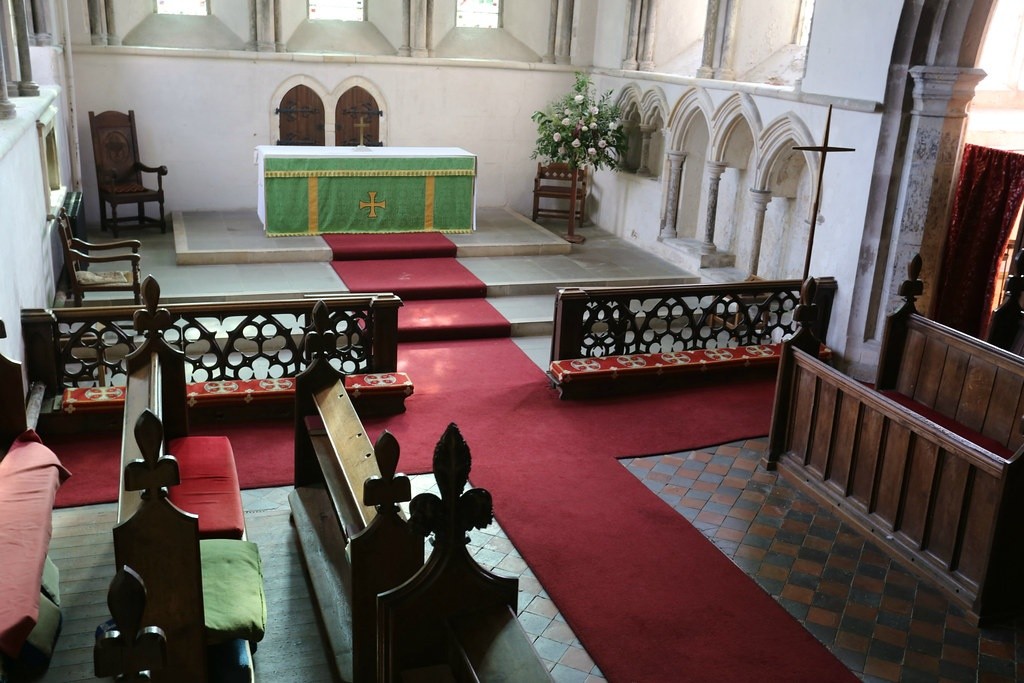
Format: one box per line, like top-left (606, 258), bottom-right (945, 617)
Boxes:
top-left (252, 146), bottom-right (478, 238)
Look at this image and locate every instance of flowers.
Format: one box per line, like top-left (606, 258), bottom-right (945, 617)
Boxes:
top-left (530, 71), bottom-right (633, 174)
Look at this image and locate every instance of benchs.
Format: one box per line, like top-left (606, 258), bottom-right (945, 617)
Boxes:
top-left (0, 254), bottom-right (1024, 683)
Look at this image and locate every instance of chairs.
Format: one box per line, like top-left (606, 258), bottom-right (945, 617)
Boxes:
top-left (533, 161), bottom-right (590, 229)
top-left (57, 208), bottom-right (144, 307)
top-left (84, 111), bottom-right (167, 236)
